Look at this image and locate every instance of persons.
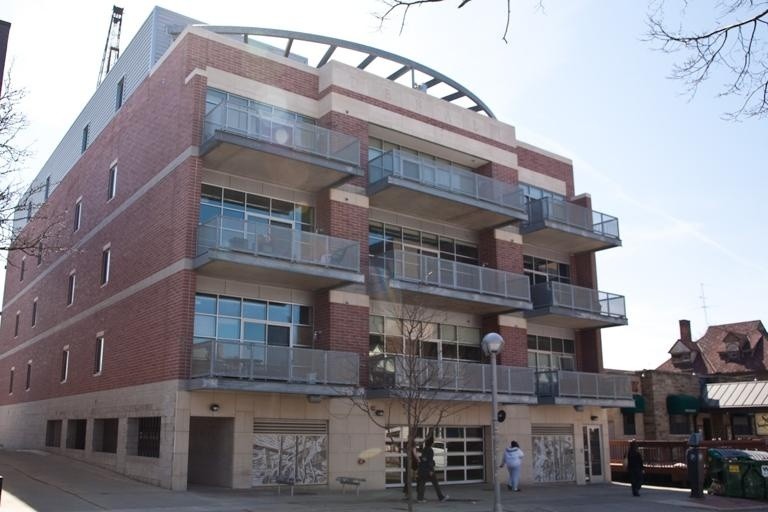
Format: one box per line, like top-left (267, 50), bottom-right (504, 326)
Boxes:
top-left (625, 443), bottom-right (645, 496)
top-left (416, 436), bottom-right (449, 502)
top-left (500, 440), bottom-right (525, 491)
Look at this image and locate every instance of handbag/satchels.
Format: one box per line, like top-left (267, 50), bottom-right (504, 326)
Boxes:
top-left (623, 458), bottom-right (629, 471)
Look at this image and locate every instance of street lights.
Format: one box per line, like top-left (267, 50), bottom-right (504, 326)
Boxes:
top-left (482, 332), bottom-right (504, 511)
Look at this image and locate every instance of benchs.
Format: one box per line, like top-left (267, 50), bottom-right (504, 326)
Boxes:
top-left (333, 474), bottom-right (368, 496)
top-left (273, 477), bottom-right (300, 498)
